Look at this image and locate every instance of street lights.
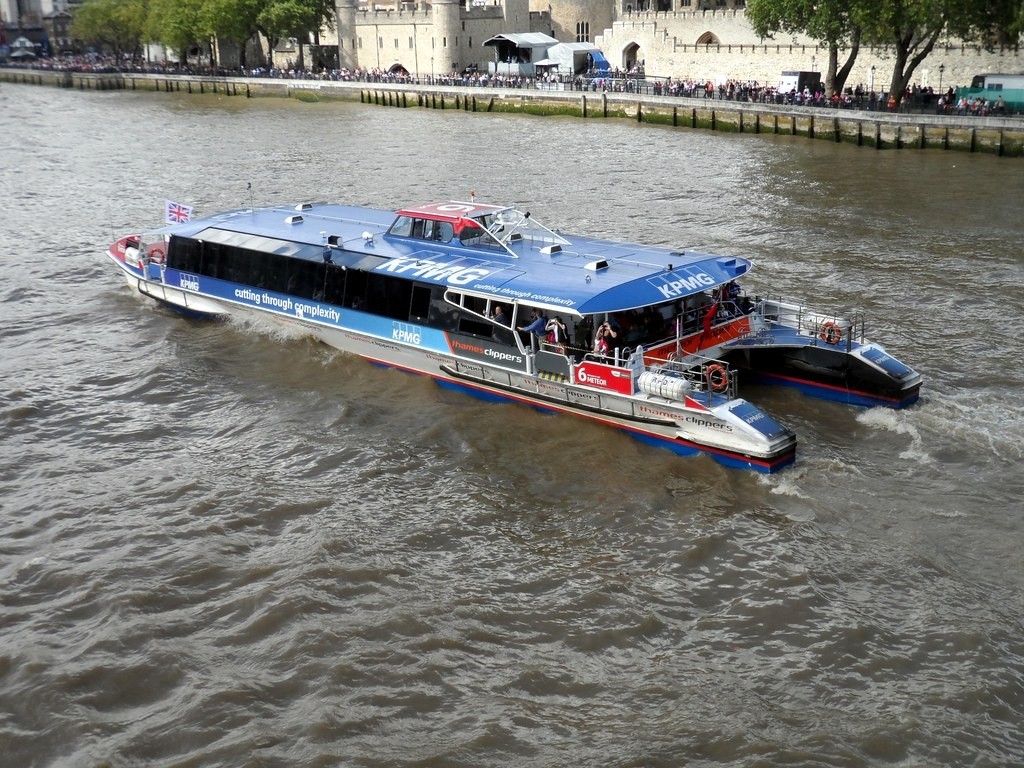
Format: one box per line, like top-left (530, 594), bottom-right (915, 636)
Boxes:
top-left (811, 56), bottom-right (815, 72)
top-left (870, 66), bottom-right (876, 93)
top-left (939, 64), bottom-right (945, 94)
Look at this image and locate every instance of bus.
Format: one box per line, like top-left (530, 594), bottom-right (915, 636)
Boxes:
top-left (955, 74), bottom-right (1024, 115)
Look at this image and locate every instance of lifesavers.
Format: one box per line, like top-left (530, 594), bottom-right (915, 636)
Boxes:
top-left (820, 322), bottom-right (843, 345)
top-left (149, 248), bottom-right (165, 265)
top-left (705, 364), bottom-right (729, 389)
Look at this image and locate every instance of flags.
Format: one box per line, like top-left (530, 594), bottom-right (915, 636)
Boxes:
top-left (166, 200), bottom-right (193, 225)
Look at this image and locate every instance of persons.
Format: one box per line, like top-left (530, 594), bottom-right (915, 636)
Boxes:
top-left (577, 315), bottom-right (594, 351)
top-left (490, 307), bottom-right (506, 343)
top-left (545, 316), bottom-right (570, 355)
top-left (531, 309), bottom-right (538, 323)
top-left (620, 279), bottom-right (765, 348)
top-left (596, 321), bottom-right (622, 367)
top-left (8, 37), bottom-right (1006, 118)
top-left (516, 310), bottom-right (548, 350)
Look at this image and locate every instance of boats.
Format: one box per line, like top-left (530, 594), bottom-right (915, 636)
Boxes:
top-left (101, 182), bottom-right (924, 483)
